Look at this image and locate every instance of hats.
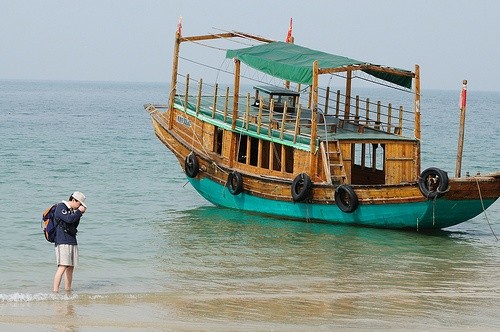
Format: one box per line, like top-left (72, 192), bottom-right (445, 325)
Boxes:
top-left (72, 192), bottom-right (87, 208)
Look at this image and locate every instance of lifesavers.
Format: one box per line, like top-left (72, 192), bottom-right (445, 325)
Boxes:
top-left (334, 185), bottom-right (359, 213)
top-left (418, 167), bottom-right (449, 200)
top-left (227, 172), bottom-right (243, 196)
top-left (184, 155), bottom-right (199, 178)
top-left (290, 172), bottom-right (312, 202)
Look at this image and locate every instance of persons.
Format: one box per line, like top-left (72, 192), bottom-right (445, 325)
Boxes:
top-left (53, 191), bottom-right (87, 294)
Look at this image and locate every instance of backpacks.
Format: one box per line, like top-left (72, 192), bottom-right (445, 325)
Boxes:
top-left (41, 204), bottom-right (66, 242)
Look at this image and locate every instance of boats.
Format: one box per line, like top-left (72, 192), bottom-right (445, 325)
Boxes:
top-left (143, 15), bottom-right (496, 232)
